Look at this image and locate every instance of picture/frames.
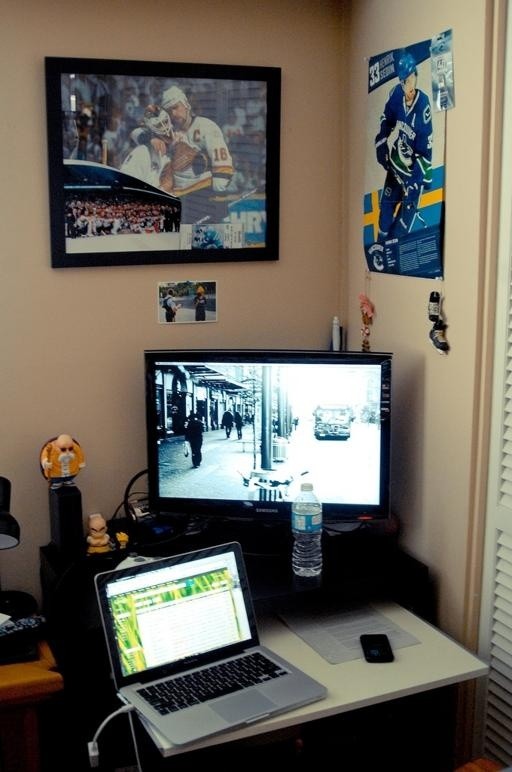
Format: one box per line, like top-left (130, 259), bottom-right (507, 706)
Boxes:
top-left (43, 54), bottom-right (283, 270)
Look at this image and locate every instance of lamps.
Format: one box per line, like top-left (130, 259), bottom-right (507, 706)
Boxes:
top-left (0, 470), bottom-right (21, 552)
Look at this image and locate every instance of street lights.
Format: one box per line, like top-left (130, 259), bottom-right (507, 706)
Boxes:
top-left (241, 377), bottom-right (257, 470)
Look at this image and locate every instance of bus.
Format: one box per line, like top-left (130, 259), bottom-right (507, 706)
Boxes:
top-left (313, 404), bottom-right (351, 439)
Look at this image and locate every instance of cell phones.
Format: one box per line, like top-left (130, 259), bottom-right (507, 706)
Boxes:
top-left (360, 634), bottom-right (394, 663)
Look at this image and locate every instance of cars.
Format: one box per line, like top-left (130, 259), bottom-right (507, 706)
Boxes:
top-left (428, 35), bottom-right (446, 53)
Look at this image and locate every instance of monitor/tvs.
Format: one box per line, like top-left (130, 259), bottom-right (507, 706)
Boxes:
top-left (144, 349), bottom-right (393, 522)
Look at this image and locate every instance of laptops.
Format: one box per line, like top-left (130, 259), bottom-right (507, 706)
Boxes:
top-left (94, 540), bottom-right (327, 748)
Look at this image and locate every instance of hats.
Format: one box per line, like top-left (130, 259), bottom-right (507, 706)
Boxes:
top-left (142, 104), bottom-right (175, 147)
top-left (196, 285), bottom-right (205, 293)
top-left (160, 86), bottom-right (192, 114)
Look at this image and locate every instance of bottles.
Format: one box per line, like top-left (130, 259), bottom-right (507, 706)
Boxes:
top-left (292, 482), bottom-right (324, 577)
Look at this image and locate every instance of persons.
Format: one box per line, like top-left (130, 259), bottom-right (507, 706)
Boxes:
top-left (164, 288), bottom-right (183, 322)
top-left (63, 197), bottom-right (180, 237)
top-left (178, 79), bottom-right (266, 195)
top-left (233, 412), bottom-right (244, 440)
top-left (184, 412), bottom-right (204, 468)
top-left (117, 104), bottom-right (174, 196)
top-left (192, 224), bottom-right (224, 249)
top-left (85, 516), bottom-right (115, 557)
top-left (129, 85), bottom-right (238, 223)
top-left (60, 73), bottom-right (168, 169)
top-left (40, 433), bottom-right (85, 490)
top-left (193, 286), bottom-right (210, 321)
top-left (374, 52), bottom-right (434, 245)
top-left (245, 413), bottom-right (251, 424)
top-left (221, 406), bottom-right (233, 437)
top-left (293, 416), bottom-right (300, 430)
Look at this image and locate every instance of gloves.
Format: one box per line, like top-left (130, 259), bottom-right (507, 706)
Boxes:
top-left (376, 145), bottom-right (390, 170)
top-left (421, 179), bottom-right (432, 190)
top-left (210, 198), bottom-right (230, 224)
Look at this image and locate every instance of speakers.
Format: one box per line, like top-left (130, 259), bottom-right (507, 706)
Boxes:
top-left (49, 485), bottom-right (83, 549)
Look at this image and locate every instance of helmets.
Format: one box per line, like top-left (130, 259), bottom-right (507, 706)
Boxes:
top-left (398, 53), bottom-right (416, 82)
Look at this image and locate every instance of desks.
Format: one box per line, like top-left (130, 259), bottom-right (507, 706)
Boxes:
top-left (1, 636), bottom-right (67, 771)
top-left (40, 511), bottom-right (495, 771)
